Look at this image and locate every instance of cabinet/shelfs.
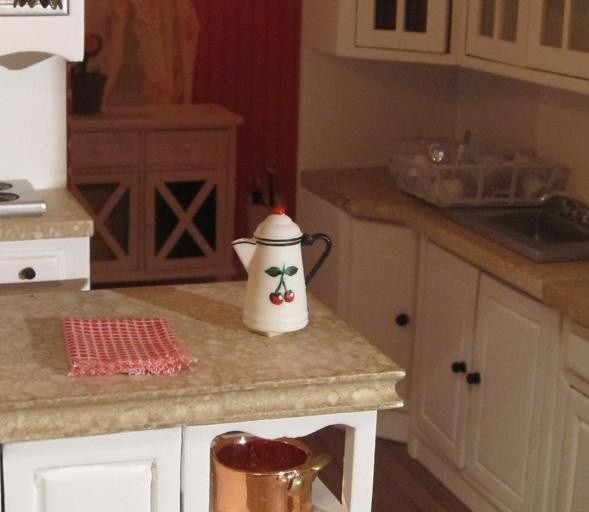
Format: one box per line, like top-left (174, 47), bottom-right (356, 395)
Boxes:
top-left (64, 101), bottom-right (245, 283)
top-left (2, 238), bottom-right (90, 295)
top-left (296, 181), bottom-right (416, 443)
top-left (307, 0), bottom-right (450, 66)
top-left (0, 1), bottom-right (83, 64)
top-left (1, 278), bottom-right (405, 511)
top-left (467, 0), bottom-right (588, 81)
top-left (416, 241), bottom-right (552, 510)
top-left (555, 315), bottom-right (588, 512)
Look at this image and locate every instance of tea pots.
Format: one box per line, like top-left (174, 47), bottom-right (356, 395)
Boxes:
top-left (230, 207), bottom-right (333, 337)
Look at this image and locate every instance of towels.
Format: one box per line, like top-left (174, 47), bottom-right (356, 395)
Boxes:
top-left (60, 314), bottom-right (199, 377)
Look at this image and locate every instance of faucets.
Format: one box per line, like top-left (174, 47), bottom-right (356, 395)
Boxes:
top-left (541, 190), bottom-right (589, 221)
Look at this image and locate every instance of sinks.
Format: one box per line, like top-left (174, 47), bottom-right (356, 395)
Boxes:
top-left (444, 205), bottom-right (589, 265)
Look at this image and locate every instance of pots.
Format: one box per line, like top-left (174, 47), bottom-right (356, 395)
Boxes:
top-left (211, 437), bottom-right (332, 512)
